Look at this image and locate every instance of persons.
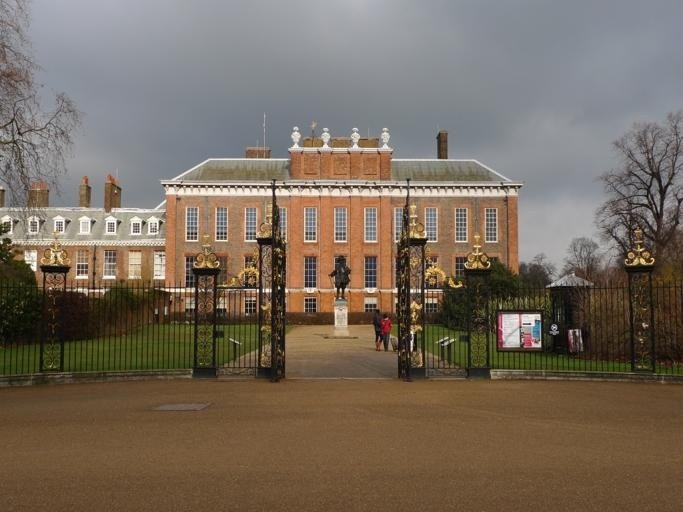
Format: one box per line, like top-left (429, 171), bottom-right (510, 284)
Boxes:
top-left (380, 314), bottom-right (392, 351)
top-left (328, 256), bottom-right (351, 300)
top-left (374, 310), bottom-right (382, 351)
